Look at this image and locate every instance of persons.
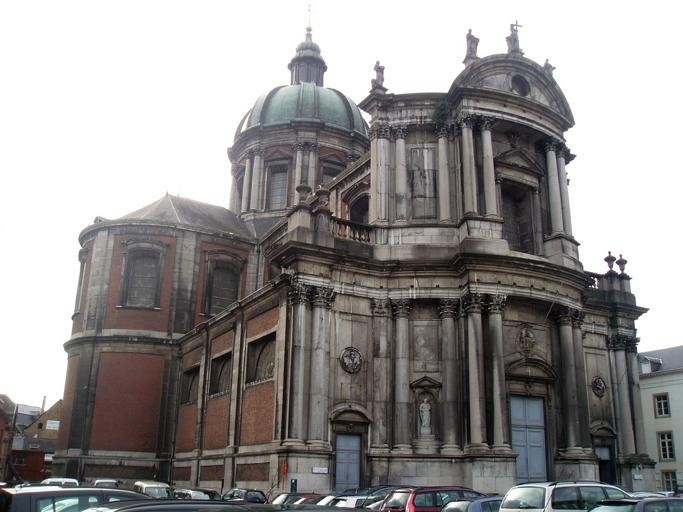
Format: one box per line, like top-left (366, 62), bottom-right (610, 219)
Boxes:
top-left (419, 398), bottom-right (431, 426)
top-left (544, 58), bottom-right (555, 74)
top-left (371, 60), bottom-right (385, 88)
top-left (507, 24), bottom-right (522, 52)
top-left (464, 29), bottom-right (479, 56)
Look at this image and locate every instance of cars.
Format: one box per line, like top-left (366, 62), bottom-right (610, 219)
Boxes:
top-left (0, 478), bottom-right (683, 512)
